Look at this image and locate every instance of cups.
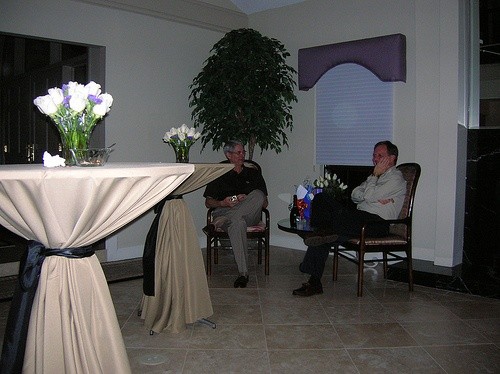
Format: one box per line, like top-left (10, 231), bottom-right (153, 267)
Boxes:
top-left (296, 216), bottom-right (306, 230)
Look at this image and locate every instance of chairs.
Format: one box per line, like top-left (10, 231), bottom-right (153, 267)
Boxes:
top-left (329, 161), bottom-right (422, 298)
top-left (200, 159), bottom-right (271, 278)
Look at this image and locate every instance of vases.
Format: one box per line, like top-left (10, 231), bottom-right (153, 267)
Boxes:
top-left (169, 140), bottom-right (195, 163)
top-left (55, 123), bottom-right (97, 166)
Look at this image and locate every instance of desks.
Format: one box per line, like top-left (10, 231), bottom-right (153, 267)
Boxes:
top-left (277, 217), bottom-right (313, 273)
top-left (148, 163), bottom-right (235, 334)
top-left (0, 164), bottom-right (195, 374)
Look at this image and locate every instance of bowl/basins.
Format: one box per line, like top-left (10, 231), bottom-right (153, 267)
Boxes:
top-left (70, 148), bottom-right (114, 165)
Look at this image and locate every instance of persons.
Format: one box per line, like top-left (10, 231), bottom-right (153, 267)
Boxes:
top-left (201, 140), bottom-right (269, 289)
top-left (292, 141), bottom-right (408, 297)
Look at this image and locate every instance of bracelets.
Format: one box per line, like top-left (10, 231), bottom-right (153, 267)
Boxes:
top-left (371, 173), bottom-right (379, 178)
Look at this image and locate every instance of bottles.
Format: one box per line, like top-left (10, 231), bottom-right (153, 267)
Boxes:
top-left (290, 194), bottom-right (299, 228)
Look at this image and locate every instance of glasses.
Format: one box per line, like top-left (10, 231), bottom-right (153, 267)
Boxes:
top-left (229, 150), bottom-right (247, 155)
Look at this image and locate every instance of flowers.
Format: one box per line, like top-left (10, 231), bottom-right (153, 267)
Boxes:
top-left (162, 124), bottom-right (201, 158)
top-left (314, 172), bottom-right (348, 190)
top-left (33, 80), bottom-right (114, 163)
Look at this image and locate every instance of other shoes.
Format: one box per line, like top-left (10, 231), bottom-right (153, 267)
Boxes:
top-left (202, 224), bottom-right (215, 237)
top-left (304, 232), bottom-right (338, 247)
top-left (234, 275), bottom-right (249, 288)
top-left (293, 283), bottom-right (323, 296)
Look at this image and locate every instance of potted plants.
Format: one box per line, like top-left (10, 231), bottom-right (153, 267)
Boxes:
top-left (187, 27), bottom-right (299, 251)
